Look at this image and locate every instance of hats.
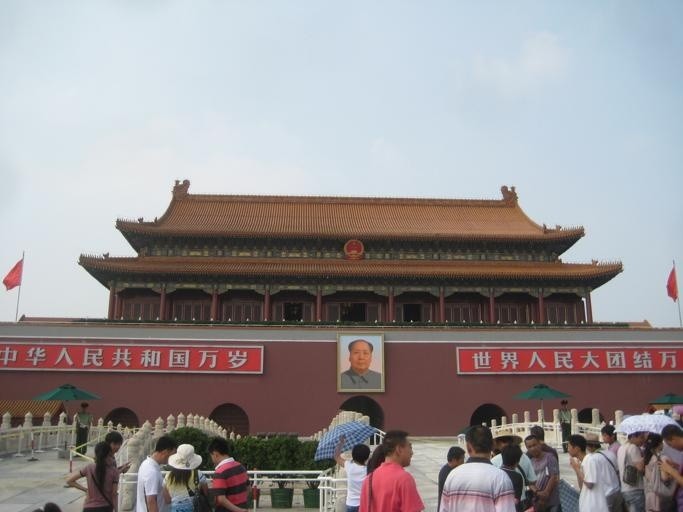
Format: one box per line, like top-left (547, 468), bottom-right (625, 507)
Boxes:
top-left (169, 443), bottom-right (203, 470)
top-left (585, 434), bottom-right (604, 446)
top-left (561, 400), bottom-right (568, 404)
top-left (81, 402), bottom-right (89, 407)
top-left (491, 431), bottom-right (522, 450)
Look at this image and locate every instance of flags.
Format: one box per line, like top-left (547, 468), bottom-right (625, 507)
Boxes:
top-left (2, 251), bottom-right (23, 325)
top-left (666, 260), bottom-right (683, 327)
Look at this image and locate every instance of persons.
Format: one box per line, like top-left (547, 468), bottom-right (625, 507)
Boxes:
top-left (558, 400), bottom-right (573, 454)
top-left (596, 424), bottom-right (621, 453)
top-left (75, 402), bottom-right (92, 457)
top-left (489, 427), bottom-right (547, 512)
top-left (525, 426), bottom-right (557, 459)
top-left (436, 446), bottom-right (465, 506)
top-left (136, 435), bottom-right (177, 512)
top-left (521, 435), bottom-right (560, 512)
top-left (641, 433), bottom-right (682, 512)
top-left (340, 339), bottom-right (381, 389)
top-left (162, 445), bottom-right (207, 511)
top-left (582, 433), bottom-right (625, 512)
top-left (207, 437), bottom-right (253, 512)
top-left (567, 434), bottom-right (608, 512)
top-left (67, 441), bottom-right (118, 512)
top-left (615, 430), bottom-right (647, 511)
top-left (661, 423), bottom-right (683, 511)
top-left (366, 445), bottom-right (386, 474)
top-left (444, 425), bottom-right (516, 511)
top-left (333, 437), bottom-right (370, 512)
top-left (494, 446), bottom-right (535, 512)
top-left (104, 431), bottom-right (132, 475)
top-left (358, 431), bottom-right (425, 511)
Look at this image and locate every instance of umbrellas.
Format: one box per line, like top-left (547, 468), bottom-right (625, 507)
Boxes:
top-left (34, 384), bottom-right (100, 446)
top-left (511, 383), bottom-right (574, 427)
top-left (311, 421), bottom-right (378, 463)
top-left (612, 414), bottom-right (683, 441)
top-left (648, 393), bottom-right (682, 410)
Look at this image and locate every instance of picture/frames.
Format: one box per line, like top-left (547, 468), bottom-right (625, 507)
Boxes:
top-left (337, 331), bottom-right (385, 393)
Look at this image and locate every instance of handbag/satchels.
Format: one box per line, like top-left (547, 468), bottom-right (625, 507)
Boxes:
top-left (193, 470), bottom-right (212, 511)
top-left (650, 455), bottom-right (677, 499)
top-left (622, 444), bottom-right (642, 487)
top-left (517, 463), bottom-right (533, 506)
